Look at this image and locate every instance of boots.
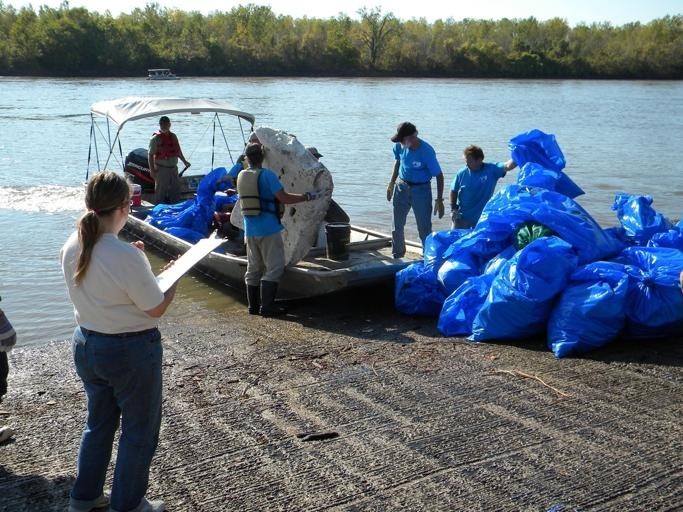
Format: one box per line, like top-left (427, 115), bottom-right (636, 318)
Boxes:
top-left (246, 285), bottom-right (259, 313)
top-left (258, 281), bottom-right (286, 316)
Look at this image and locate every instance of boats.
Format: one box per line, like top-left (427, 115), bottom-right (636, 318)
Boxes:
top-left (82, 96), bottom-right (426, 304)
top-left (145, 69), bottom-right (180, 81)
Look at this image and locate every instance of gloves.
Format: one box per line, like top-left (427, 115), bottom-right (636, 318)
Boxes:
top-left (387, 182), bottom-right (394, 201)
top-left (306, 190), bottom-right (324, 201)
top-left (434, 199), bottom-right (444, 218)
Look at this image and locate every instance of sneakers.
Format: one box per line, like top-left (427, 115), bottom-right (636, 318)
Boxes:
top-left (68, 490), bottom-right (112, 512)
top-left (109, 497), bottom-right (164, 512)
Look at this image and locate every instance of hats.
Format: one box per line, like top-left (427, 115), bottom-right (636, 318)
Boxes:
top-left (160, 116), bottom-right (169, 123)
top-left (308, 147), bottom-right (323, 158)
top-left (245, 143), bottom-right (261, 154)
top-left (391, 122), bottom-right (416, 141)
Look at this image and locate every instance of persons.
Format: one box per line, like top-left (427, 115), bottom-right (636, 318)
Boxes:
top-left (448, 144), bottom-right (518, 230)
top-left (243, 143), bottom-right (323, 316)
top-left (386, 121), bottom-right (445, 259)
top-left (0, 308), bottom-right (17, 442)
top-left (59, 170), bottom-right (183, 512)
top-left (305, 144), bottom-right (324, 159)
top-left (146, 115), bottom-right (191, 204)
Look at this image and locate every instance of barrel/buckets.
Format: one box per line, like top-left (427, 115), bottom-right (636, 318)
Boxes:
top-left (324, 222), bottom-right (351, 260)
top-left (130, 184), bottom-right (142, 205)
